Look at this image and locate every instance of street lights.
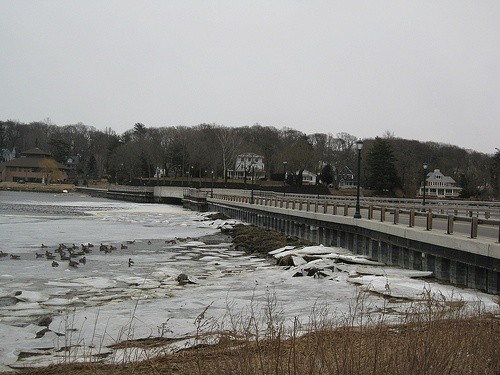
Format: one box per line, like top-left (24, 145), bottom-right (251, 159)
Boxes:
top-left (191, 165), bottom-right (194, 188)
top-left (250, 161), bottom-right (257, 204)
top-left (423, 162), bottom-right (427, 212)
top-left (352, 140), bottom-right (363, 218)
top-left (283, 161), bottom-right (288, 199)
top-left (210, 168), bottom-right (214, 198)
top-left (204, 169), bottom-right (207, 191)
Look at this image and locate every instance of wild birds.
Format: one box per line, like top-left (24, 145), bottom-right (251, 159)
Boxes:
top-left (10, 254), bottom-right (21, 259)
top-left (0, 251), bottom-right (8, 257)
top-left (35, 237), bottom-right (200, 269)
